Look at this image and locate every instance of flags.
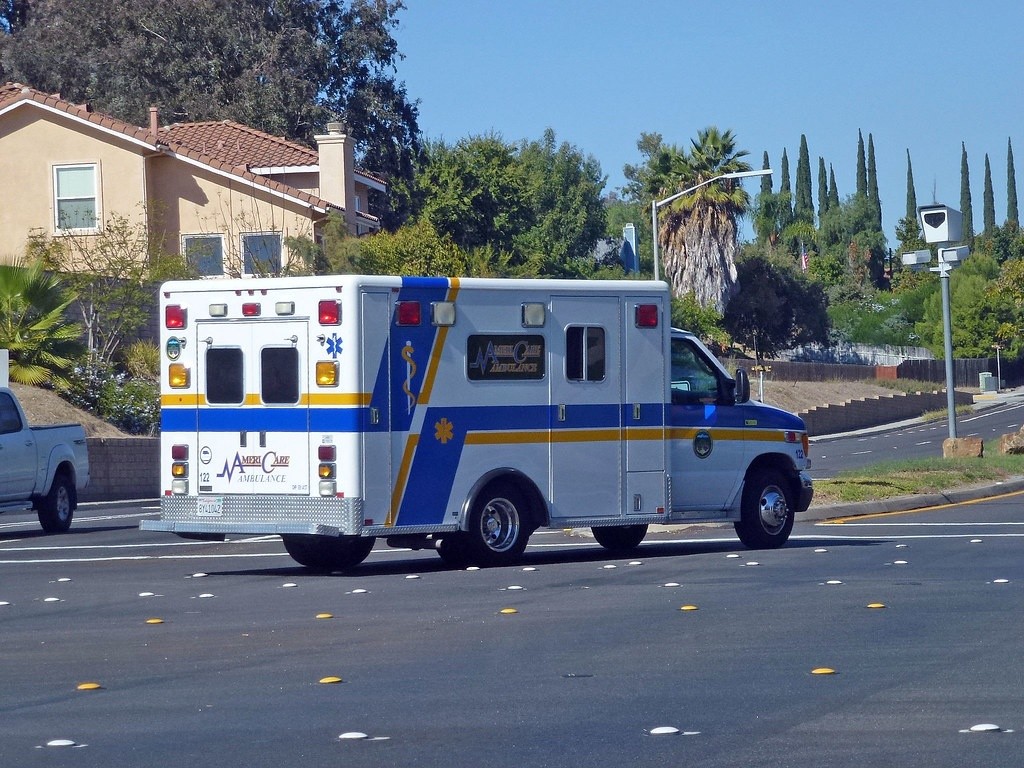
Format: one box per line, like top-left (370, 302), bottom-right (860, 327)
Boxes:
top-left (801, 244), bottom-right (808, 273)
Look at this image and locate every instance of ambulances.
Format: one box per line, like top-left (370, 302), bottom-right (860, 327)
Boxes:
top-left (134, 273), bottom-right (814, 576)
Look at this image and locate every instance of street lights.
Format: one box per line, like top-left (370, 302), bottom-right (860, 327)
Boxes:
top-left (651, 168), bottom-right (773, 280)
top-left (990, 345), bottom-right (1004, 392)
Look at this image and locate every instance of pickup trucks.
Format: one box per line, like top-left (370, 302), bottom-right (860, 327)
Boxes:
top-left (0, 386), bottom-right (92, 534)
